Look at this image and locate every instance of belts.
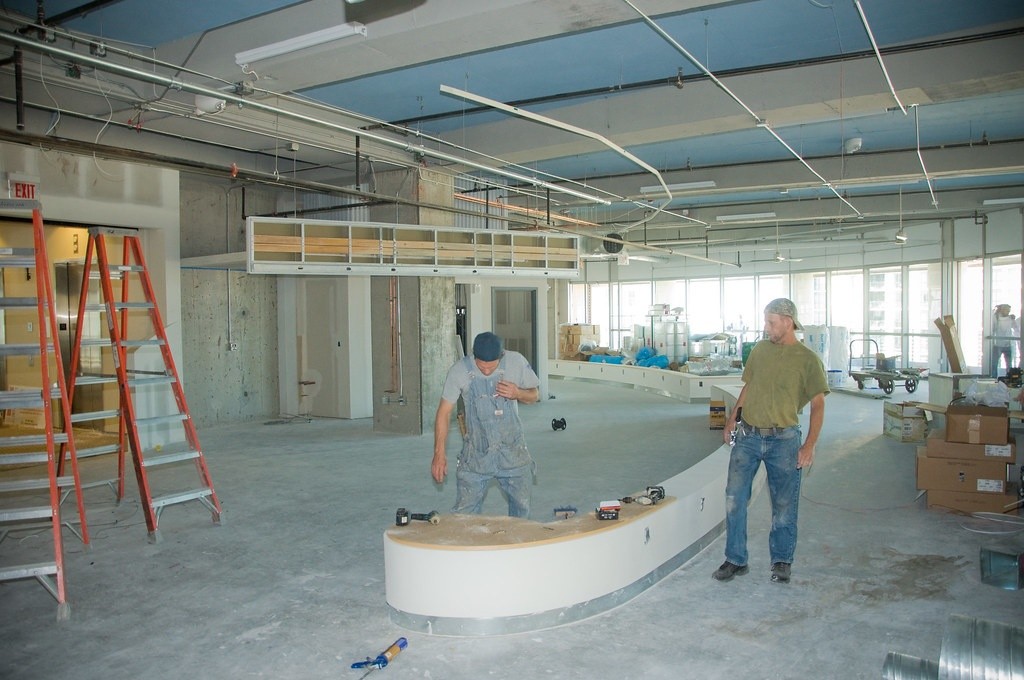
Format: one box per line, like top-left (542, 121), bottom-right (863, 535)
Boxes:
top-left (742, 418), bottom-right (787, 436)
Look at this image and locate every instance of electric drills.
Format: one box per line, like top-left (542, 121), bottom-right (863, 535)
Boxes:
top-left (395, 507), bottom-right (440, 528)
top-left (618, 485), bottom-right (665, 506)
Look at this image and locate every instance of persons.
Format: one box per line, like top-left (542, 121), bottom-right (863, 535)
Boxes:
top-left (711, 298), bottom-right (830, 583)
top-left (431, 332), bottom-right (541, 519)
top-left (993, 304), bottom-right (1021, 378)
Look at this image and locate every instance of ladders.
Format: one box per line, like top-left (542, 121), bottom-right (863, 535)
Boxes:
top-left (0, 195), bottom-right (92, 618)
top-left (50, 224), bottom-right (224, 544)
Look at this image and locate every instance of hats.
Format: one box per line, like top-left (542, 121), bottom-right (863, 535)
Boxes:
top-left (473, 332), bottom-right (503, 362)
top-left (764, 298), bottom-right (805, 331)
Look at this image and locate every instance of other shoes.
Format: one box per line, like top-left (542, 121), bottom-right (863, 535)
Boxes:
top-left (771, 562), bottom-right (791, 584)
top-left (712, 561), bottom-right (749, 582)
